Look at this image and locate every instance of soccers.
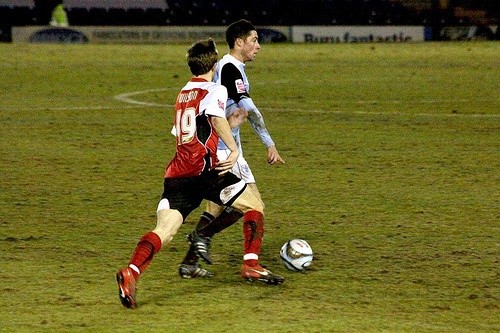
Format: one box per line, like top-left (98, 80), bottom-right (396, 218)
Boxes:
top-left (278, 238), bottom-right (315, 273)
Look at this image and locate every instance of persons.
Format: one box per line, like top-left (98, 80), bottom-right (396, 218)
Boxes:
top-left (115, 37), bottom-right (286, 309)
top-left (177, 18), bottom-right (287, 277)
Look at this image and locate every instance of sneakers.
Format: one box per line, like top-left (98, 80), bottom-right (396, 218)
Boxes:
top-left (116, 268), bottom-right (139, 310)
top-left (179, 263), bottom-right (215, 281)
top-left (241, 264), bottom-right (286, 285)
top-left (187, 230), bottom-right (215, 265)
